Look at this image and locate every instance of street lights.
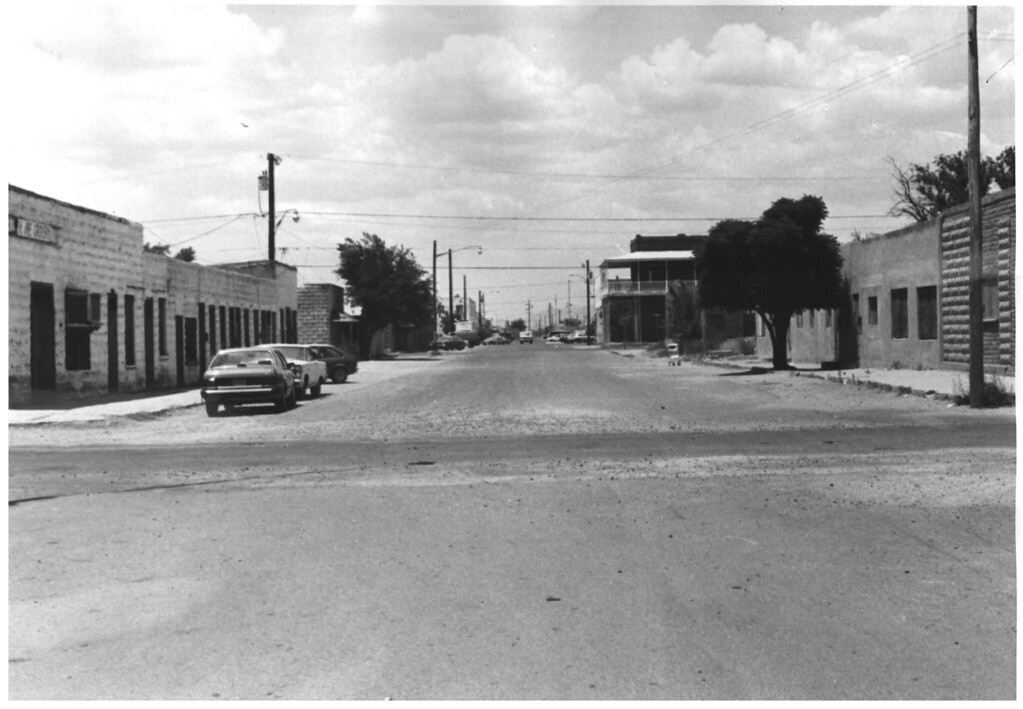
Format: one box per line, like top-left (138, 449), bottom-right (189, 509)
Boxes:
top-left (268, 209), bottom-right (300, 261)
top-left (432, 246), bottom-right (482, 345)
top-left (570, 273), bottom-right (591, 345)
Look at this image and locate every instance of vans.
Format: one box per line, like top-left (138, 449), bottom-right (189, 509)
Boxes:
top-left (519, 331), bottom-right (532, 343)
top-left (568, 330), bottom-right (595, 343)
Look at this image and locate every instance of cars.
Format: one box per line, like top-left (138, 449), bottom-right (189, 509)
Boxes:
top-left (262, 343), bottom-right (327, 400)
top-left (546, 335), bottom-right (560, 341)
top-left (484, 334), bottom-right (507, 344)
top-left (200, 345), bottom-right (297, 416)
top-left (306, 345), bottom-right (359, 384)
